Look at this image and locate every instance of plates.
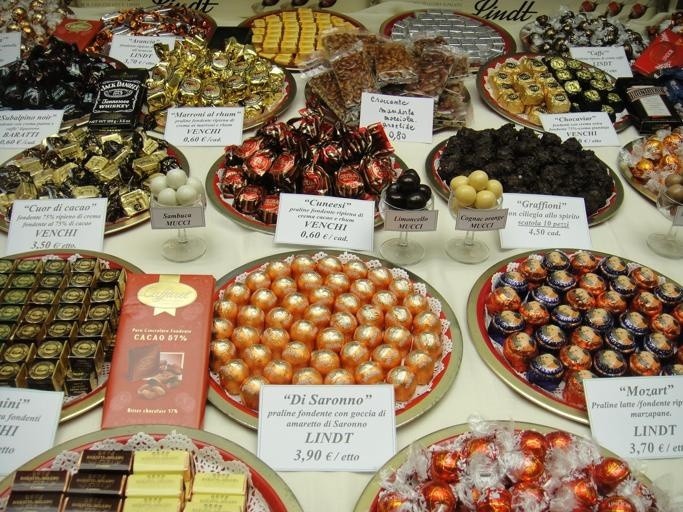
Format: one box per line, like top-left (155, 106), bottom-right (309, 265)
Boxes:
top-left (615, 137), bottom-right (672, 206)
top-left (1, 53), bottom-right (129, 134)
top-left (205, 152), bottom-right (408, 236)
top-left (378, 7), bottom-right (517, 75)
top-left (91, 10), bottom-right (218, 54)
top-left (467, 247), bottom-right (682, 424)
top-left (0, 423), bottom-right (305, 512)
top-left (302, 73), bottom-right (471, 133)
top-left (427, 126), bottom-right (623, 231)
top-left (519, 19), bottom-right (648, 62)
top-left (354, 416), bottom-right (673, 510)
top-left (140, 54), bottom-right (294, 134)
top-left (241, 9), bottom-right (368, 73)
top-left (476, 53), bottom-right (630, 134)
top-left (1, 133), bottom-right (191, 239)
top-left (204, 249), bottom-right (463, 433)
top-left (0, 248), bottom-right (145, 424)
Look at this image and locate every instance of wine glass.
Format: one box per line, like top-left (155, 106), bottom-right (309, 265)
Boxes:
top-left (151, 182), bottom-right (208, 261)
top-left (442, 192), bottom-right (506, 264)
top-left (645, 179), bottom-right (681, 261)
top-left (376, 183), bottom-right (437, 265)
